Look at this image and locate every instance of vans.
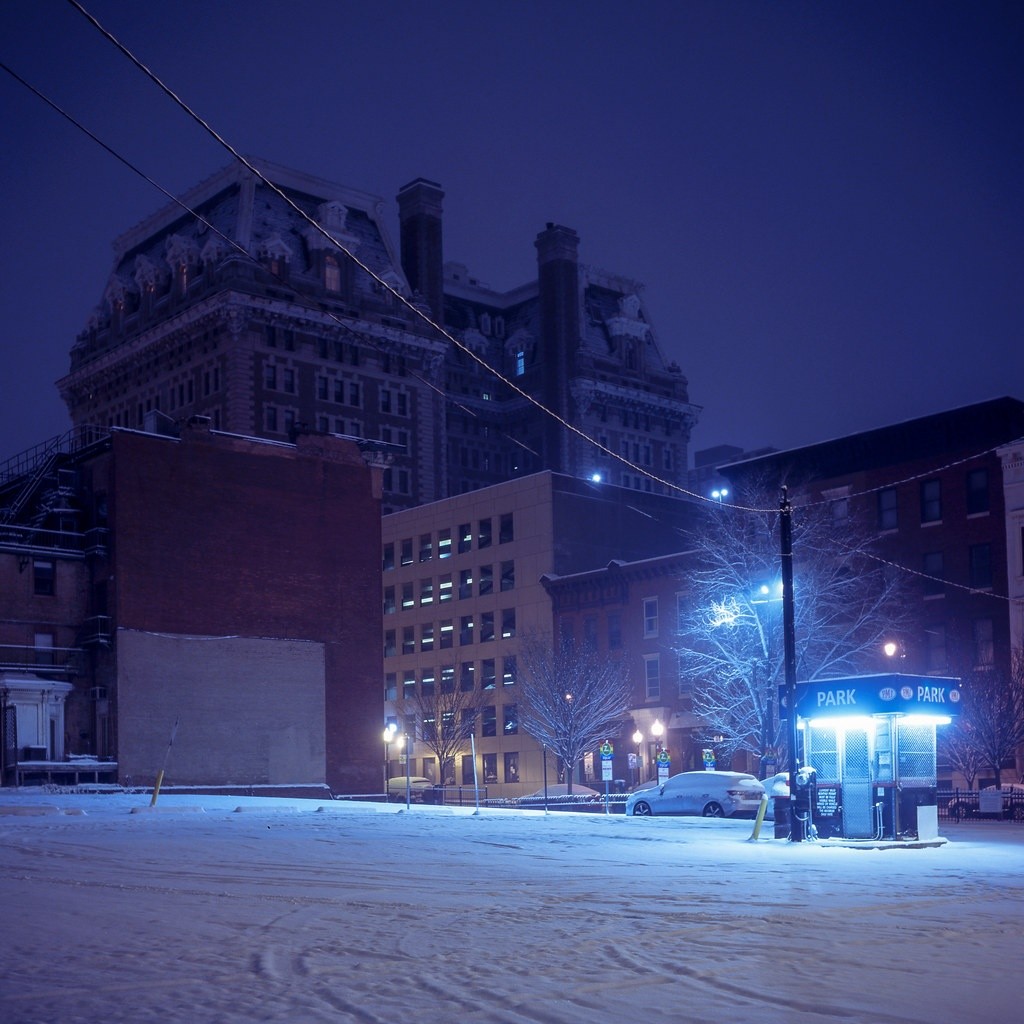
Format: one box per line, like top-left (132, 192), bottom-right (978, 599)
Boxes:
top-left (388, 777), bottom-right (435, 804)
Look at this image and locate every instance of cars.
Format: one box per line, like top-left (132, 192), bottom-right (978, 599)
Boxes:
top-left (948, 783), bottom-right (1024, 820)
top-left (517, 783), bottom-right (606, 812)
top-left (625, 771), bottom-right (767, 818)
top-left (759, 772), bottom-right (795, 821)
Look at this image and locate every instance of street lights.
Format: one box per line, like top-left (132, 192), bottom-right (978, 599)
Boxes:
top-left (396, 732), bottom-right (410, 806)
top-left (650, 718), bottom-right (665, 788)
top-left (632, 728), bottom-right (642, 786)
top-left (383, 727), bottom-right (392, 797)
top-left (712, 489), bottom-right (728, 510)
top-left (762, 584), bottom-right (785, 778)
top-left (884, 641), bottom-right (898, 673)
